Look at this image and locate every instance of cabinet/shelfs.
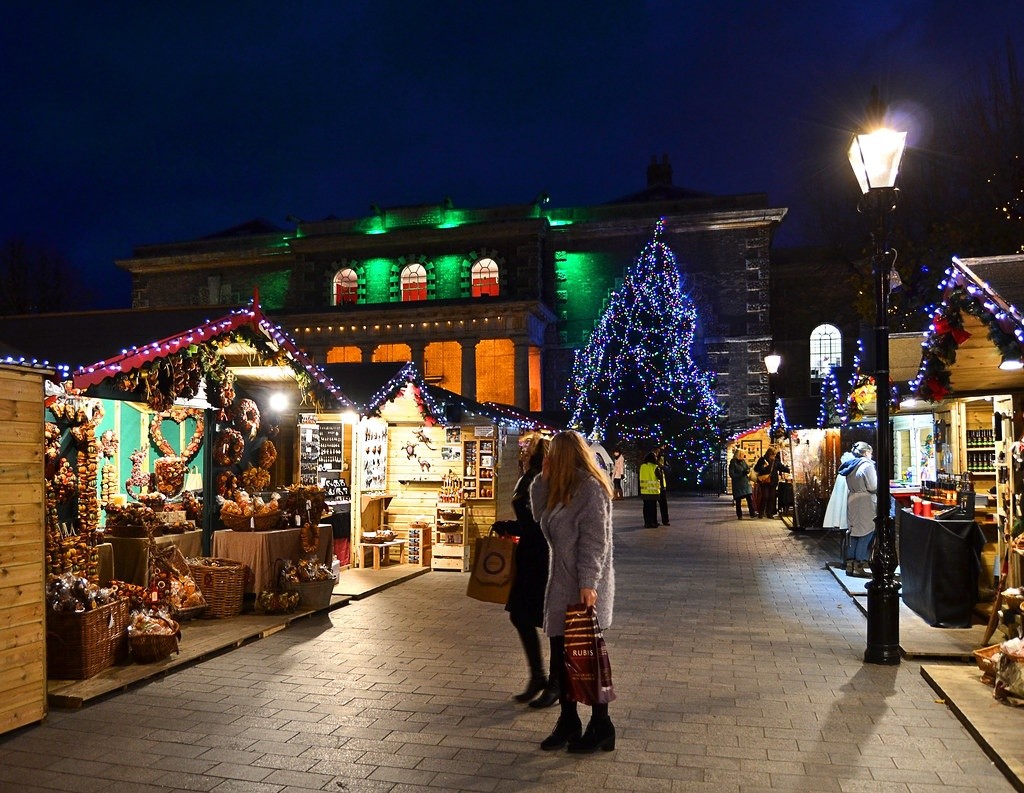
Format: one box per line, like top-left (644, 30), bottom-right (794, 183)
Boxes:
top-left (430, 507), bottom-right (470, 571)
top-left (960, 404), bottom-right (997, 476)
top-left (463, 438), bottom-right (497, 500)
top-left (408, 527), bottom-right (430, 565)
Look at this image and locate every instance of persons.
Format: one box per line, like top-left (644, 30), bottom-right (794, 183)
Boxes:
top-left (639, 446), bottom-right (671, 528)
top-left (753, 438), bottom-right (791, 518)
top-left (728, 449), bottom-right (760, 519)
top-left (490, 434), bottom-right (561, 708)
top-left (609, 449), bottom-right (625, 500)
top-left (823, 441), bottom-right (877, 578)
top-left (529, 431), bottom-right (616, 753)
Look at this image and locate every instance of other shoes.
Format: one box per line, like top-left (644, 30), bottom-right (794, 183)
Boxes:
top-left (738, 511), bottom-right (774, 520)
top-left (657, 522), bottom-right (660, 525)
top-left (662, 522), bottom-right (671, 526)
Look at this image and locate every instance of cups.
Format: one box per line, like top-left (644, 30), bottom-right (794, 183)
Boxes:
top-left (914, 498), bottom-right (922, 514)
top-left (922, 501), bottom-right (931, 517)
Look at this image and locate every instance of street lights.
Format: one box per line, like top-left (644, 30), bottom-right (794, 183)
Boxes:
top-left (846, 90), bottom-right (909, 669)
top-left (764, 350), bottom-right (781, 444)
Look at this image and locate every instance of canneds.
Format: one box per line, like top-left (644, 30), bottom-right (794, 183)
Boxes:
top-left (325, 478), bottom-right (350, 501)
top-left (441, 442), bottom-right (493, 503)
top-left (919, 480), bottom-right (958, 505)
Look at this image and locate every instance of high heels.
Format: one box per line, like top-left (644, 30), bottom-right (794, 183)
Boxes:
top-left (529, 674), bottom-right (563, 708)
top-left (541, 713), bottom-right (582, 750)
top-left (568, 716), bottom-right (615, 753)
top-left (516, 672), bottom-right (549, 703)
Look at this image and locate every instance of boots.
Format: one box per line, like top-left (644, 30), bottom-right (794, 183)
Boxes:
top-left (853, 560), bottom-right (873, 579)
top-left (845, 559), bottom-right (853, 576)
top-left (612, 489), bottom-right (624, 501)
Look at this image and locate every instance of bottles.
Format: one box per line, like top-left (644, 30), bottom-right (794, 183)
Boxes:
top-left (966, 429), bottom-right (995, 472)
top-left (920, 481), bottom-right (957, 505)
top-left (467, 462), bottom-right (476, 477)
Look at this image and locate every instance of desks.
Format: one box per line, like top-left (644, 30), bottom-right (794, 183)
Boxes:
top-left (212, 523), bottom-right (332, 604)
top-left (94, 543), bottom-right (116, 587)
top-left (900, 509), bottom-right (978, 626)
top-left (102, 529), bottom-right (207, 589)
top-left (358, 539), bottom-right (404, 570)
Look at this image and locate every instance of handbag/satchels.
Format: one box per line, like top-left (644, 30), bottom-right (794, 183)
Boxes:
top-left (466, 524), bottom-right (518, 604)
top-left (563, 603), bottom-right (616, 706)
top-left (758, 474), bottom-right (772, 485)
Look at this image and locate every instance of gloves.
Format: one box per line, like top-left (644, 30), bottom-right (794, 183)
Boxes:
top-left (492, 521), bottom-right (508, 536)
top-left (621, 474), bottom-right (625, 480)
top-left (609, 472), bottom-right (613, 477)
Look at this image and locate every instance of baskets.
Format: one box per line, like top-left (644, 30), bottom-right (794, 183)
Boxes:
top-left (408, 522), bottom-right (430, 528)
top-left (361, 532), bottom-right (398, 544)
top-left (188, 556), bottom-right (245, 619)
top-left (220, 494), bottom-right (282, 532)
top-left (258, 557), bottom-right (301, 615)
top-left (280, 490), bottom-right (326, 526)
top-left (437, 513), bottom-right (463, 532)
top-left (45, 594), bottom-right (129, 679)
top-left (128, 615), bottom-right (179, 662)
